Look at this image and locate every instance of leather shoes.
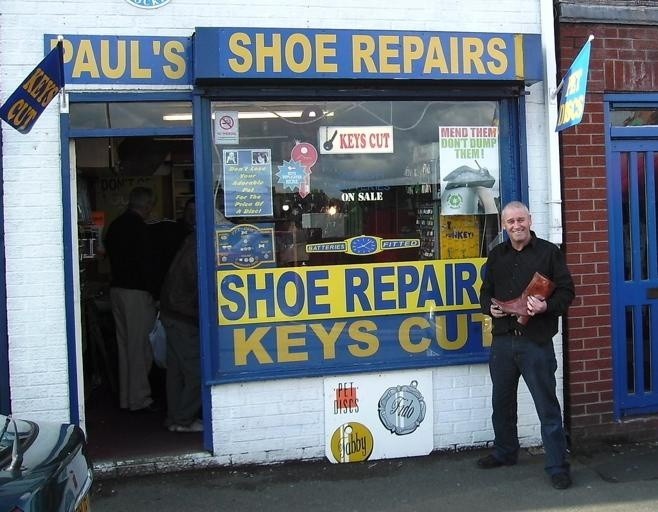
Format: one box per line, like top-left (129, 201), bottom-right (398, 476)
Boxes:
top-left (121, 401), bottom-right (204, 433)
top-left (551, 471), bottom-right (571, 489)
top-left (477, 454), bottom-right (514, 469)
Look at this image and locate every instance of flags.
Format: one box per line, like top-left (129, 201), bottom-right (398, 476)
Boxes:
top-left (556, 36), bottom-right (596, 132)
top-left (0, 41), bottom-right (67, 135)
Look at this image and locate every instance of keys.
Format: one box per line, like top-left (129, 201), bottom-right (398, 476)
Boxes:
top-left (300, 105), bottom-right (325, 149)
top-left (323, 131), bottom-right (338, 151)
top-left (290, 141), bottom-right (319, 202)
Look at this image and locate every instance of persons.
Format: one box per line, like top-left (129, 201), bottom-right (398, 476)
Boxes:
top-left (159, 227), bottom-right (204, 433)
top-left (104, 186), bottom-right (168, 415)
top-left (173, 197), bottom-right (196, 234)
top-left (478, 200), bottom-right (577, 490)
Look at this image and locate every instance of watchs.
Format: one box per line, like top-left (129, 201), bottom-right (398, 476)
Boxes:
top-left (303, 231), bottom-right (422, 259)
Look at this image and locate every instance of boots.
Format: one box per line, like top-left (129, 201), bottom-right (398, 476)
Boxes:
top-left (490, 271), bottom-right (557, 326)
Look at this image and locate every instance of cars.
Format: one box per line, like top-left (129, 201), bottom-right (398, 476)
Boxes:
top-left (0, 407), bottom-right (98, 512)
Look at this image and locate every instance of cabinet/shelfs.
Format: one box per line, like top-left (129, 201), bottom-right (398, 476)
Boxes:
top-left (171, 163), bottom-right (231, 221)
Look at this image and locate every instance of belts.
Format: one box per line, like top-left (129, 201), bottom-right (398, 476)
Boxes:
top-left (512, 328), bottom-right (522, 337)
